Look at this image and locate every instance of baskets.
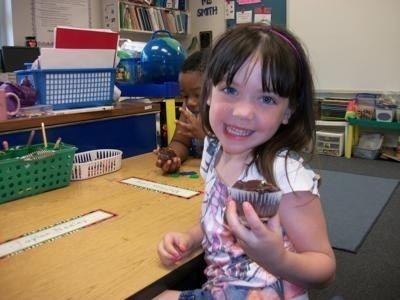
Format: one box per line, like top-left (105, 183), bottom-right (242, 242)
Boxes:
top-left (0, 142), bottom-right (74, 204)
top-left (70, 148), bottom-right (123, 181)
top-left (16, 67), bottom-right (117, 109)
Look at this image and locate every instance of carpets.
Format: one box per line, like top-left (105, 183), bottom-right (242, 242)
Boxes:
top-left (311, 167), bottom-right (400, 254)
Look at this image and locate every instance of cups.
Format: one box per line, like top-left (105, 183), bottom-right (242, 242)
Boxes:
top-left (0, 88), bottom-right (21, 121)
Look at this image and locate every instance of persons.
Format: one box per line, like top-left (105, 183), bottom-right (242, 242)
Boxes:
top-left (156, 22), bottom-right (338, 300)
top-left (152, 46), bottom-right (210, 175)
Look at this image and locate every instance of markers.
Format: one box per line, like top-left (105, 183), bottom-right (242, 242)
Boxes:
top-left (72, 159), bottom-right (115, 178)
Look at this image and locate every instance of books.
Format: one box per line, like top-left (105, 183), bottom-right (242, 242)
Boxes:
top-left (311, 95), bottom-right (352, 121)
top-left (120, 0), bottom-right (188, 34)
top-left (39, 25), bottom-right (118, 110)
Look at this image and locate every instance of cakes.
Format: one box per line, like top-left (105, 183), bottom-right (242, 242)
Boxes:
top-left (158, 147), bottom-right (177, 163)
top-left (228, 179), bottom-right (283, 217)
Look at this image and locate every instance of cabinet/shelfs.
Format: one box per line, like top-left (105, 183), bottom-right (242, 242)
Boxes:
top-left (102, 0), bottom-right (191, 38)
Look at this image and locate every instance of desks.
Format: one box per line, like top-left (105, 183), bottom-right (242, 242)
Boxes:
top-left (344, 117), bottom-right (400, 160)
top-left (314, 119), bottom-right (349, 154)
top-left (0, 146), bottom-right (206, 300)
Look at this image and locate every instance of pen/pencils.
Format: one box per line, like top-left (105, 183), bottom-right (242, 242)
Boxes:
top-left (2, 140), bottom-right (8, 151)
top-left (41, 122), bottom-right (48, 148)
top-left (28, 131), bottom-right (35, 145)
top-left (53, 137), bottom-right (62, 150)
